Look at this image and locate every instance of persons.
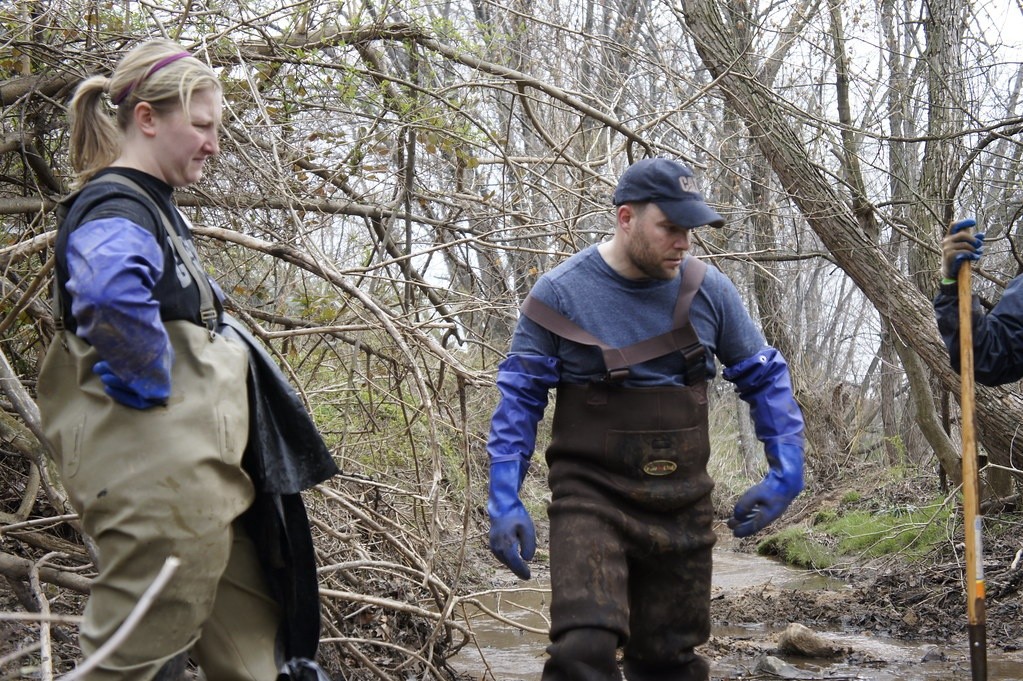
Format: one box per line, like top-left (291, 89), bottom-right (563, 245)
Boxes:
top-left (36, 38), bottom-right (338, 680)
top-left (933, 218), bottom-right (1023, 388)
top-left (485, 159), bottom-right (807, 680)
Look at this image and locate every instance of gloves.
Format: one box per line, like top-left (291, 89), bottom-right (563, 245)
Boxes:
top-left (942, 219), bottom-right (985, 281)
top-left (722, 348), bottom-right (805, 537)
top-left (486, 352), bottom-right (560, 581)
top-left (65, 217), bottom-right (173, 410)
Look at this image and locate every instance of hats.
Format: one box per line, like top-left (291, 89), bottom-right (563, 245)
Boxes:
top-left (612, 155), bottom-right (725, 229)
